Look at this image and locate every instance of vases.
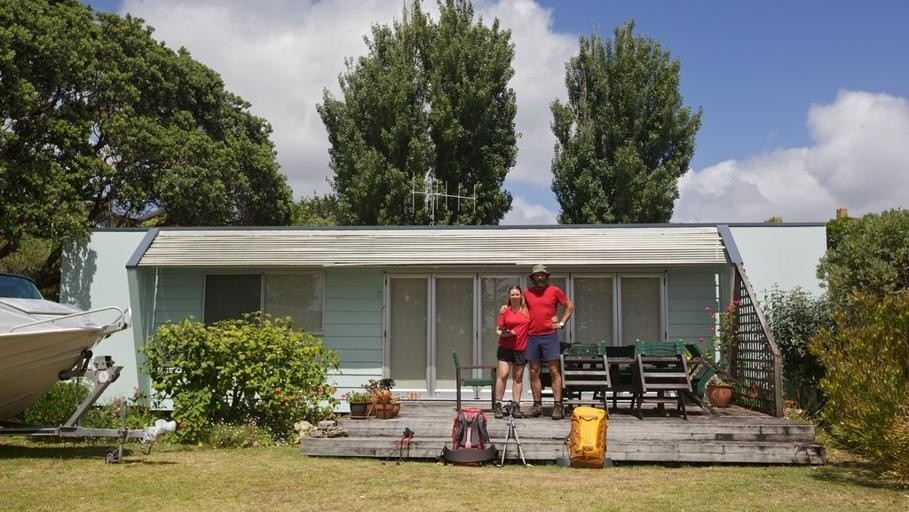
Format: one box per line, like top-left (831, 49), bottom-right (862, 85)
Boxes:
top-left (707, 384), bottom-right (734, 408)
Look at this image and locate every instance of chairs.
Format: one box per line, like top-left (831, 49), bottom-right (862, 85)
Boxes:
top-left (560, 340), bottom-right (742, 420)
top-left (452, 352), bottom-right (499, 411)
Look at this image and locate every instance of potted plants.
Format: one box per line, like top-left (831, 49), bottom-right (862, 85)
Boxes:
top-left (347, 378), bottom-right (402, 419)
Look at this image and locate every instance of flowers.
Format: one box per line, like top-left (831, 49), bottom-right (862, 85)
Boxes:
top-left (699, 300), bottom-right (742, 385)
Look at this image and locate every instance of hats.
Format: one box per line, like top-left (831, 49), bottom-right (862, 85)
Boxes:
top-left (527, 264), bottom-right (552, 279)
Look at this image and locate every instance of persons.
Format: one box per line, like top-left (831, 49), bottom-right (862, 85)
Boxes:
top-left (499, 263), bottom-right (574, 419)
top-left (494, 285), bottom-right (559, 419)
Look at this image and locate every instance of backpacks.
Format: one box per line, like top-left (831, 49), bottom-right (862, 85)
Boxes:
top-left (569, 404), bottom-right (609, 468)
top-left (449, 408), bottom-right (491, 466)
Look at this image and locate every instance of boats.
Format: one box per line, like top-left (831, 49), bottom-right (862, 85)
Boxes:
top-left (0, 271), bottom-right (125, 423)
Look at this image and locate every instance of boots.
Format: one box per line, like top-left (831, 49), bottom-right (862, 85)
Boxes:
top-left (552, 400), bottom-right (564, 420)
top-left (493, 401), bottom-right (504, 419)
top-left (511, 401), bottom-right (522, 419)
top-left (531, 401), bottom-right (543, 417)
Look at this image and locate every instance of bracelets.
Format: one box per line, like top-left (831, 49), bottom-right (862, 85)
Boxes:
top-left (560, 321), bottom-right (563, 327)
